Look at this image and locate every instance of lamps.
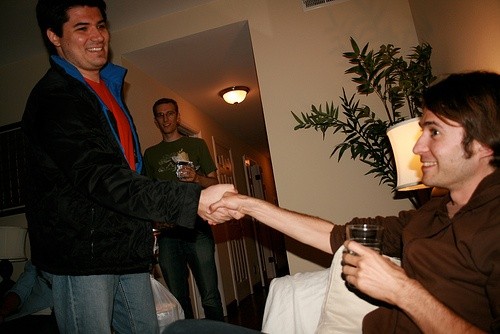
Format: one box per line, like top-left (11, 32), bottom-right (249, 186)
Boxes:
top-left (386, 116), bottom-right (436, 192)
top-left (217, 85), bottom-right (251, 105)
top-left (0, 227), bottom-right (27, 287)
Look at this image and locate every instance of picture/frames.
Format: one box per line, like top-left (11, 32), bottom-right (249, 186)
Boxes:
top-left (0, 121), bottom-right (28, 217)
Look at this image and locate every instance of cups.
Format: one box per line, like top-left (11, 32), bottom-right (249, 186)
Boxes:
top-left (346, 225), bottom-right (383, 254)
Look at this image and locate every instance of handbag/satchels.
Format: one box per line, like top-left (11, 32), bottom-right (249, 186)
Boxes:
top-left (149, 273), bottom-right (185, 334)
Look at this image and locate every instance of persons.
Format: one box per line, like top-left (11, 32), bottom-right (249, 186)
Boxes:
top-left (18, 0), bottom-right (244, 334)
top-left (162, 70), bottom-right (500, 334)
top-left (139, 97), bottom-right (225, 324)
